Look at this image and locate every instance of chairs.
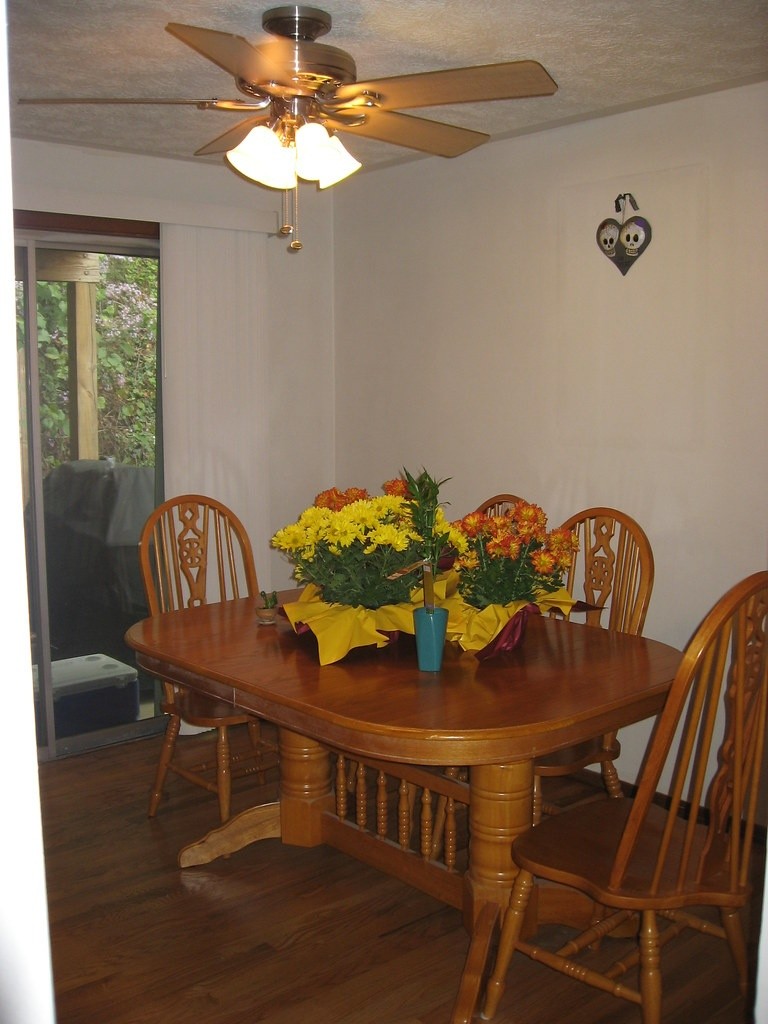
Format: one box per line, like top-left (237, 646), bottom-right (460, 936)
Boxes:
top-left (138, 493), bottom-right (283, 860)
top-left (481, 568), bottom-right (768, 1024)
top-left (425, 506), bottom-right (656, 875)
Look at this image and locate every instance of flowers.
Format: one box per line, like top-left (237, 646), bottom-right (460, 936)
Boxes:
top-left (270, 478), bottom-right (468, 611)
top-left (453, 499), bottom-right (580, 610)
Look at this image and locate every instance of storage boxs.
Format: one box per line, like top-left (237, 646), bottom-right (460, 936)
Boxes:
top-left (32, 651), bottom-right (138, 753)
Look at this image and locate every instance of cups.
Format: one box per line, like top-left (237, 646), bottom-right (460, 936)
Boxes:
top-left (412, 607), bottom-right (448, 672)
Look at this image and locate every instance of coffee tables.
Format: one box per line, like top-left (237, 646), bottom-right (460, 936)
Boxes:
top-left (124, 587), bottom-right (689, 1024)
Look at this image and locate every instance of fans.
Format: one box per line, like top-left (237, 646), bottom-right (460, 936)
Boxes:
top-left (17, 6), bottom-right (558, 158)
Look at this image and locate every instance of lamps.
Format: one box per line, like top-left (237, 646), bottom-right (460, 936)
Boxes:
top-left (225, 115), bottom-right (362, 254)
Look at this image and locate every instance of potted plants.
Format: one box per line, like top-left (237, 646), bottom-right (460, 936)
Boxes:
top-left (399, 462), bottom-right (453, 672)
